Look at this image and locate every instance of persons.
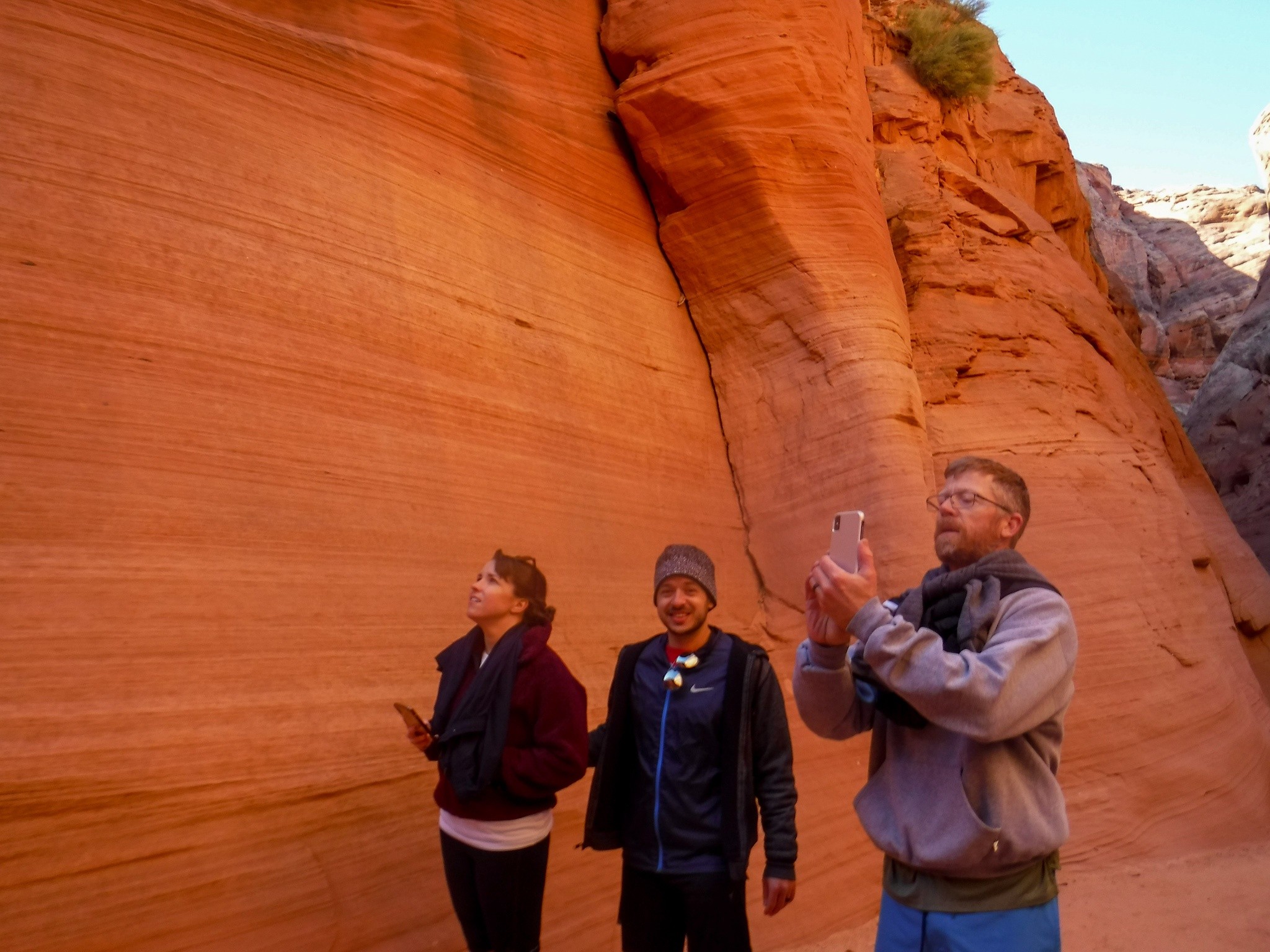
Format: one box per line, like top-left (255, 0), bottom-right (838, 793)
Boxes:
top-left (791, 454), bottom-right (1078, 952)
top-left (574, 544), bottom-right (798, 952)
top-left (407, 549), bottom-right (587, 952)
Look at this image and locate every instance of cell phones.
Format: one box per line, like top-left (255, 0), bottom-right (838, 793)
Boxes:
top-left (829, 510), bottom-right (865, 573)
top-left (393, 702), bottom-right (434, 739)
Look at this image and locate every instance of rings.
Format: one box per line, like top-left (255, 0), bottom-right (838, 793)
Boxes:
top-left (812, 583), bottom-right (820, 592)
top-left (414, 730), bottom-right (420, 736)
top-left (786, 898), bottom-right (790, 902)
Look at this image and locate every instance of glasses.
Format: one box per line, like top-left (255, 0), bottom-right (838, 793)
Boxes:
top-left (926, 493), bottom-right (1012, 514)
top-left (662, 651), bottom-right (700, 691)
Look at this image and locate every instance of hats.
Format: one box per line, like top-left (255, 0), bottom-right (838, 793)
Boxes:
top-left (653, 543), bottom-right (717, 612)
